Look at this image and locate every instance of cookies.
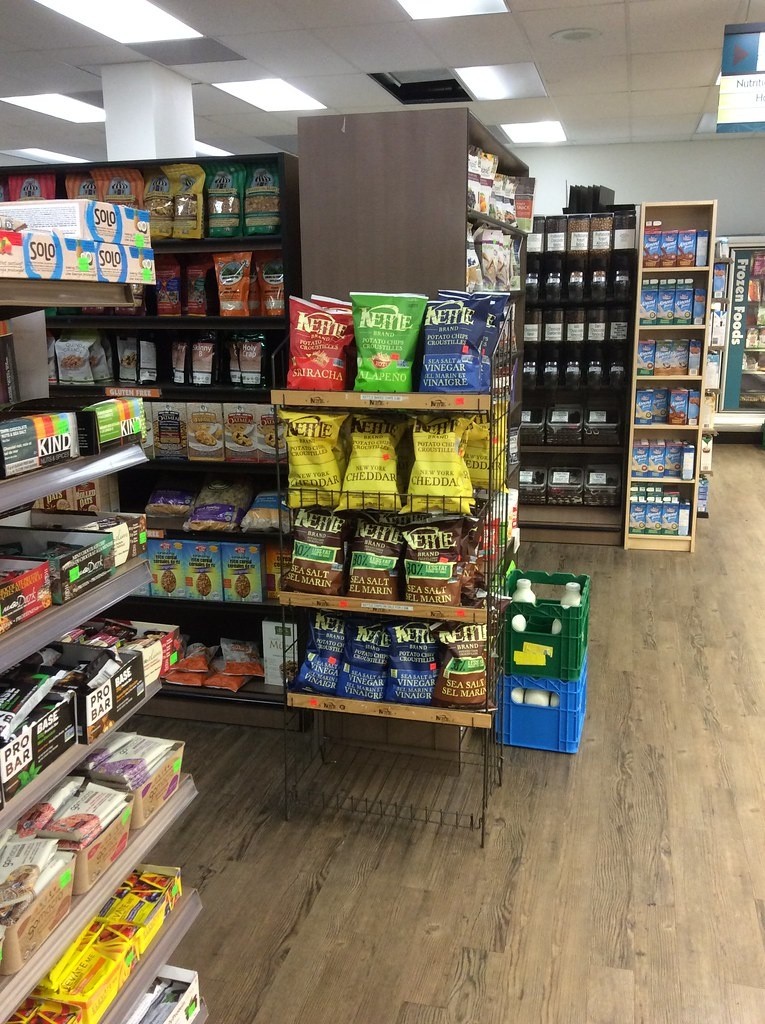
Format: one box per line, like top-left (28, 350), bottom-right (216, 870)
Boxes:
top-left (232, 431), bottom-right (252, 446)
top-left (196, 573), bottom-right (211, 596)
top-left (161, 571), bottom-right (176, 593)
top-left (195, 432), bottom-right (217, 445)
top-left (88, 505), bottom-right (97, 511)
top-left (57, 500), bottom-right (70, 509)
top-left (266, 433), bottom-right (285, 449)
top-left (235, 576), bottom-right (250, 598)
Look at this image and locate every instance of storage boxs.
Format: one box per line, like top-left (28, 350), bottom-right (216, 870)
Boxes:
top-left (0, 198), bottom-right (157, 285)
top-left (70, 792), bottom-right (136, 894)
top-left (0, 690), bottom-right (79, 803)
top-left (0, 557), bottom-right (52, 637)
top-left (0, 411), bottom-right (80, 480)
top-left (53, 642), bottom-right (146, 747)
top-left (0, 508), bottom-right (148, 567)
top-left (83, 621), bottom-right (184, 688)
top-left (0, 852), bottom-right (202, 1024)
top-left (0, 526), bottom-right (116, 606)
top-left (496, 568), bottom-right (592, 681)
top-left (129, 740), bottom-right (185, 831)
top-left (0, 394), bottom-right (148, 458)
top-left (517, 212), bottom-right (730, 536)
top-left (492, 648), bottom-right (588, 754)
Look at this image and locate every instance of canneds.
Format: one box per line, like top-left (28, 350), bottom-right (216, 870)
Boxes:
top-left (523, 362), bottom-right (624, 375)
top-left (526, 270), bottom-right (629, 285)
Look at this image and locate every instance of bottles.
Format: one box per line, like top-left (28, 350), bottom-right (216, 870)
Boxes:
top-left (511, 579), bottom-right (582, 633)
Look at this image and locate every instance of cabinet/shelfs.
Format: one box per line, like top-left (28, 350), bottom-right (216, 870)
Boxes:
top-left (514, 206), bottom-right (640, 547)
top-left (0, 151), bottom-right (311, 734)
top-left (297, 106), bottom-right (533, 776)
top-left (696, 258), bottom-right (734, 519)
top-left (0, 278), bottom-right (209, 1024)
top-left (623, 198), bottom-right (718, 552)
top-left (270, 305), bottom-right (513, 849)
